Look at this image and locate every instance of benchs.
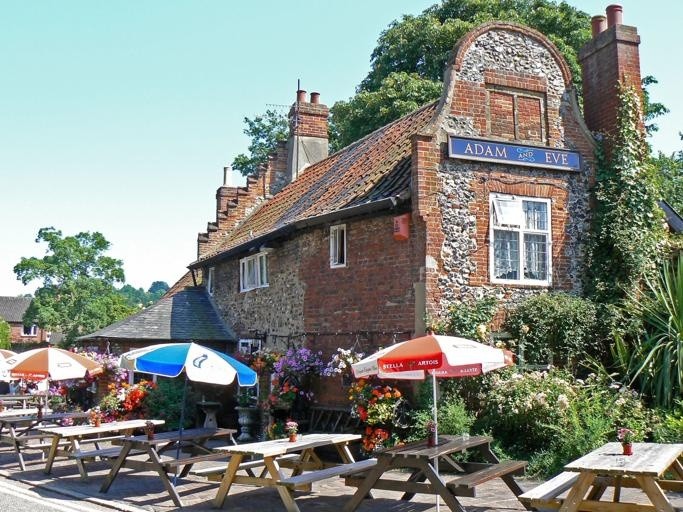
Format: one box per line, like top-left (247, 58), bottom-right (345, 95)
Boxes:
top-left (446, 460), bottom-right (527, 490)
top-left (279, 457), bottom-right (389, 488)
top-left (2, 423), bottom-right (148, 457)
top-left (517, 473), bottom-right (583, 504)
top-left (159, 453), bottom-right (297, 478)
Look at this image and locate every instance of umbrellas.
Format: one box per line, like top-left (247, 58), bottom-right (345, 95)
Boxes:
top-left (117, 341), bottom-right (257, 487)
top-left (2, 345), bottom-right (105, 425)
top-left (0, 348), bottom-right (18, 383)
top-left (349, 330), bottom-right (515, 512)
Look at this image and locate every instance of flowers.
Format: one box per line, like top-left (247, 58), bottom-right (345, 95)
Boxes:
top-left (223, 347), bottom-right (370, 443)
top-left (426, 421), bottom-right (437, 434)
top-left (617, 427), bottom-right (635, 442)
top-left (29, 346), bottom-right (156, 422)
top-left (350, 378), bottom-right (403, 452)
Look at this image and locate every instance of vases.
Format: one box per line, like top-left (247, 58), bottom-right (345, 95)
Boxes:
top-left (428, 435), bottom-right (433, 446)
top-left (624, 442), bottom-right (632, 454)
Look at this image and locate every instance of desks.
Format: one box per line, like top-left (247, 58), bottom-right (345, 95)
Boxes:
top-left (210, 434), bottom-right (365, 511)
top-left (2, 412), bottom-right (105, 473)
top-left (36, 419), bottom-right (165, 483)
top-left (339, 434), bottom-right (531, 512)
top-left (91, 428), bottom-right (238, 507)
